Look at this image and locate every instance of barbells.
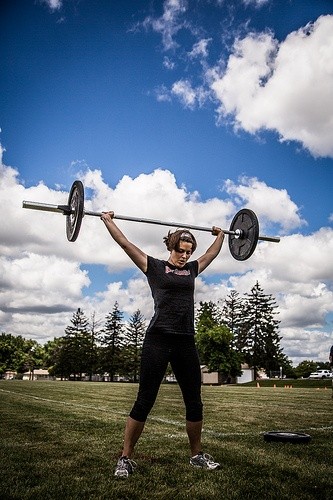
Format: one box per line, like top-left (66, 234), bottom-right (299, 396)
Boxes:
top-left (22, 180), bottom-right (280, 261)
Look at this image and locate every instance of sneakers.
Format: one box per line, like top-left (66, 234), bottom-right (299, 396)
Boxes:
top-left (114, 455), bottom-right (137, 478)
top-left (189, 453), bottom-right (220, 471)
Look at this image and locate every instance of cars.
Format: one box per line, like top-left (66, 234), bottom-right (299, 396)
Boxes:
top-left (310, 370), bottom-right (333, 379)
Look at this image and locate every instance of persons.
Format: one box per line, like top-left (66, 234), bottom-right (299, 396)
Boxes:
top-left (101, 211), bottom-right (226, 477)
